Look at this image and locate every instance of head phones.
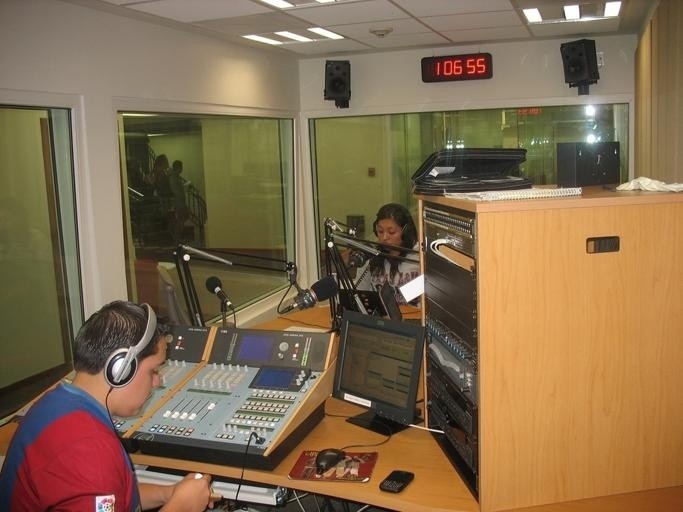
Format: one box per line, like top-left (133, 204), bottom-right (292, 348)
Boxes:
top-left (372, 203), bottom-right (417, 249)
top-left (102, 302), bottom-right (156, 387)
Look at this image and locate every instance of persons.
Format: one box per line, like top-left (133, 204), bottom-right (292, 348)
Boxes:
top-left (353, 203), bottom-right (422, 292)
top-left (128, 136), bottom-right (151, 183)
top-left (0, 299), bottom-right (222, 512)
top-left (153, 154), bottom-right (173, 218)
top-left (168, 159), bottom-right (188, 224)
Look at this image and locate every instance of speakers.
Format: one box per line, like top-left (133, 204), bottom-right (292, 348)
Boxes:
top-left (556, 142), bottom-right (598, 187)
top-left (559, 39), bottom-right (599, 84)
top-left (593, 141), bottom-right (620, 185)
top-left (323, 60), bottom-right (350, 101)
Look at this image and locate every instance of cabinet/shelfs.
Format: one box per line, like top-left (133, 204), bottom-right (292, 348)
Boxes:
top-left (415, 183), bottom-right (683, 511)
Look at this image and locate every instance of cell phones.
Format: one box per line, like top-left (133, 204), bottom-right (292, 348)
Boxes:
top-left (379, 470), bottom-right (414, 492)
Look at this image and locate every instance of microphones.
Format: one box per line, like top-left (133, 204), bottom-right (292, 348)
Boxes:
top-left (205, 276), bottom-right (234, 310)
top-left (293, 275), bottom-right (337, 311)
top-left (351, 243), bottom-right (382, 266)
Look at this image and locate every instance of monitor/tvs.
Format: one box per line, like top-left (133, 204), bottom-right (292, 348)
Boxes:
top-left (331, 310), bottom-right (426, 436)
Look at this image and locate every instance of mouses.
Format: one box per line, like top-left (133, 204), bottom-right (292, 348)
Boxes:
top-left (315, 448), bottom-right (343, 472)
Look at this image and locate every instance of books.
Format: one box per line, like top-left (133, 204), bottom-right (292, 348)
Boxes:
top-left (443, 187), bottom-right (583, 202)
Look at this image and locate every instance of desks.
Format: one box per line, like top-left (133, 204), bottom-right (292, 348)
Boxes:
top-left (246, 293), bottom-right (421, 336)
top-left (1, 350), bottom-right (682, 511)
